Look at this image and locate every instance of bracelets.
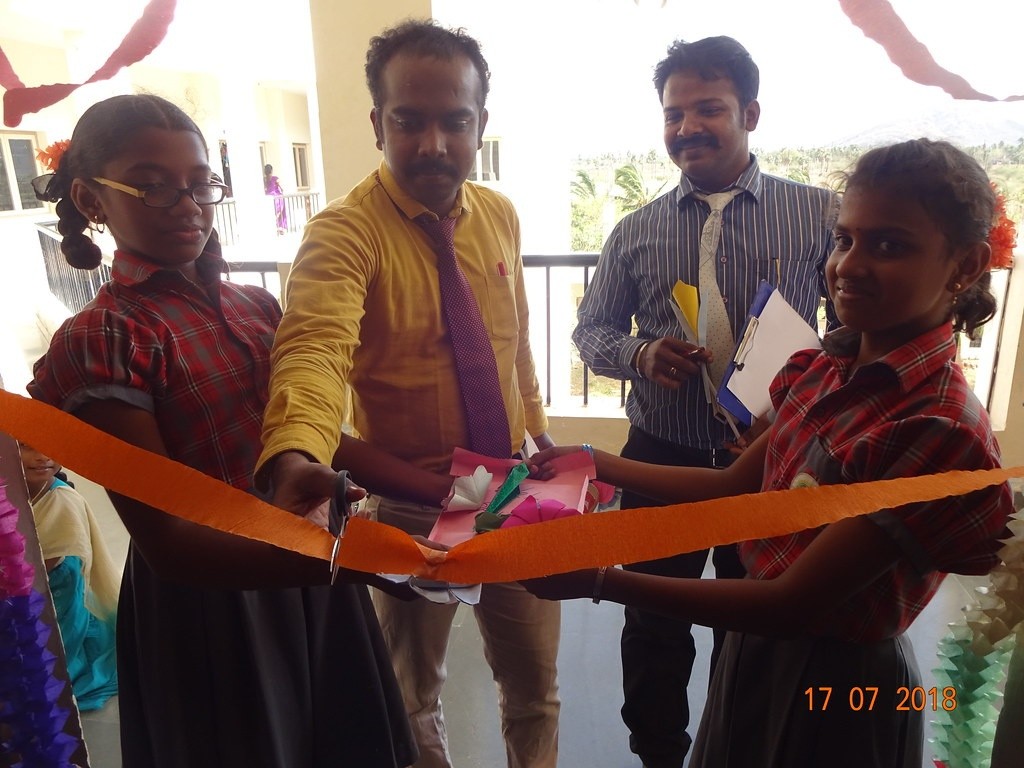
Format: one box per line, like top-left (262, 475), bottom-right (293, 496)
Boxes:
top-left (581, 443), bottom-right (593, 456)
top-left (634, 342), bottom-right (649, 379)
top-left (593, 567), bottom-right (606, 605)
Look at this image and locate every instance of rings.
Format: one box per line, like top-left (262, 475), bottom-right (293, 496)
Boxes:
top-left (668, 368), bottom-right (676, 376)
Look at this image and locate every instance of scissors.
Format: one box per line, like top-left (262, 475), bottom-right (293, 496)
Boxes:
top-left (330, 470), bottom-right (353, 585)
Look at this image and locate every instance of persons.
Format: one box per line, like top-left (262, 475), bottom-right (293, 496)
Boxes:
top-left (475, 138), bottom-right (1013, 768)
top-left (27, 93), bottom-right (455, 768)
top-left (251, 18), bottom-right (560, 768)
top-left (263, 164), bottom-right (287, 235)
top-left (570, 35), bottom-right (842, 768)
top-left (16, 440), bottom-right (122, 713)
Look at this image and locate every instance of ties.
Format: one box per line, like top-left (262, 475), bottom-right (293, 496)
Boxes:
top-left (694, 190), bottom-right (743, 426)
top-left (418, 214), bottom-right (514, 460)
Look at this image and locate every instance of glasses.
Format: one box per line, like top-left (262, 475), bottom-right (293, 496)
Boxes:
top-left (92, 177), bottom-right (231, 211)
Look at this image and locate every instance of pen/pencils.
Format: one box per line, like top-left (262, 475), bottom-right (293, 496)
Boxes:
top-left (680, 347), bottom-right (705, 358)
